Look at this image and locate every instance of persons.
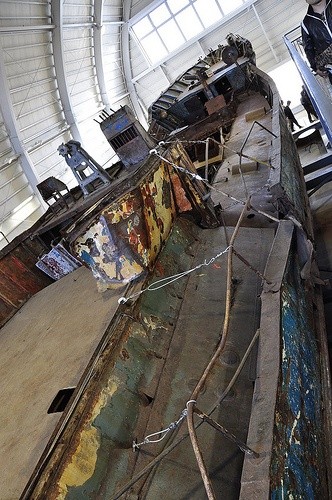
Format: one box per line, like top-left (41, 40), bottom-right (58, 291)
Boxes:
top-left (284, 100), bottom-right (304, 132)
top-left (281, 100), bottom-right (285, 110)
top-left (301, 84), bottom-right (314, 108)
top-left (300, 92), bottom-right (319, 124)
top-left (300, 0), bottom-right (332, 86)
